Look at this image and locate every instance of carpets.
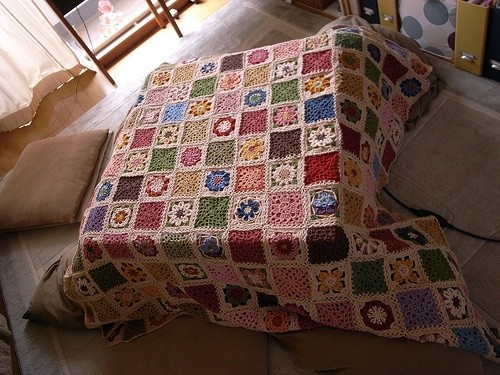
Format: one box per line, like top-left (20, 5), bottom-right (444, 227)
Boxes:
top-left (381, 96), bottom-right (498, 244)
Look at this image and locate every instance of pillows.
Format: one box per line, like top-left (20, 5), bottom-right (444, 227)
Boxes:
top-left (1, 130), bottom-right (112, 229)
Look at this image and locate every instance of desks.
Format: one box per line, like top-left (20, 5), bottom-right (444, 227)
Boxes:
top-left (60, 16), bottom-right (499, 364)
